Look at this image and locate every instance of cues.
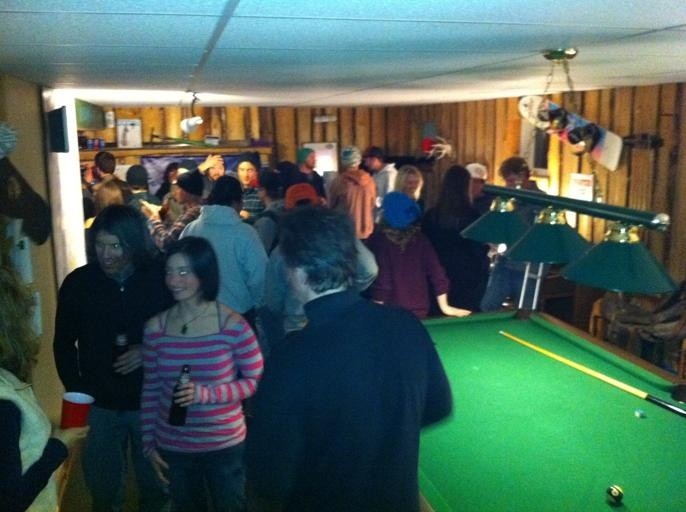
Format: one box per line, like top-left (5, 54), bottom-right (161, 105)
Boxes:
top-left (498, 330), bottom-right (686, 417)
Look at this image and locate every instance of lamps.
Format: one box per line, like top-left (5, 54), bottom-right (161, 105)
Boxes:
top-left (179, 89), bottom-right (204, 134)
top-left (459, 48), bottom-right (675, 295)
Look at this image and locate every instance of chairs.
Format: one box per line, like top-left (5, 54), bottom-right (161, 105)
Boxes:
top-left (590, 281), bottom-right (686, 376)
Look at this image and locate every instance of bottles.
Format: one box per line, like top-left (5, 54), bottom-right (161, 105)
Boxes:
top-left (164, 364), bottom-right (192, 428)
top-left (113, 330), bottom-right (130, 355)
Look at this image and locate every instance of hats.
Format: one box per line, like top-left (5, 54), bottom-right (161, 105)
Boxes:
top-left (176, 168), bottom-right (203, 196)
top-left (285, 183), bottom-right (318, 210)
top-left (464, 163), bottom-right (488, 181)
top-left (238, 152), bottom-right (260, 171)
top-left (383, 191), bottom-right (423, 231)
top-left (297, 148), bottom-right (314, 163)
top-left (362, 147), bottom-right (382, 158)
top-left (341, 146), bottom-right (362, 167)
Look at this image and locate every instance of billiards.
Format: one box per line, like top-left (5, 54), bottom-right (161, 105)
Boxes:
top-left (635, 409), bottom-right (644, 418)
top-left (607, 485), bottom-right (623, 503)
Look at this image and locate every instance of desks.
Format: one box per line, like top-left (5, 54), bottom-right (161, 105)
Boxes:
top-left (403, 308), bottom-right (686, 512)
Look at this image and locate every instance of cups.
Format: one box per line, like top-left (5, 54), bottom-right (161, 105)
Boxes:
top-left (58, 392), bottom-right (95, 430)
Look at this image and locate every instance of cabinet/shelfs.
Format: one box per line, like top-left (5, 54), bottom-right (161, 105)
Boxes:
top-left (79, 139), bottom-right (272, 166)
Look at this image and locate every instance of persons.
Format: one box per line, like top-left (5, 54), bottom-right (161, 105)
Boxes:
top-left (0, 243), bottom-right (90, 512)
top-left (241, 203), bottom-right (454, 511)
top-left (139, 236), bottom-right (265, 511)
top-left (52, 204), bottom-right (175, 511)
top-left (80, 147), bottom-right (551, 356)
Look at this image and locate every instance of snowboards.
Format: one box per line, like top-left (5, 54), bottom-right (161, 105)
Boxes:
top-left (517, 96), bottom-right (623, 171)
